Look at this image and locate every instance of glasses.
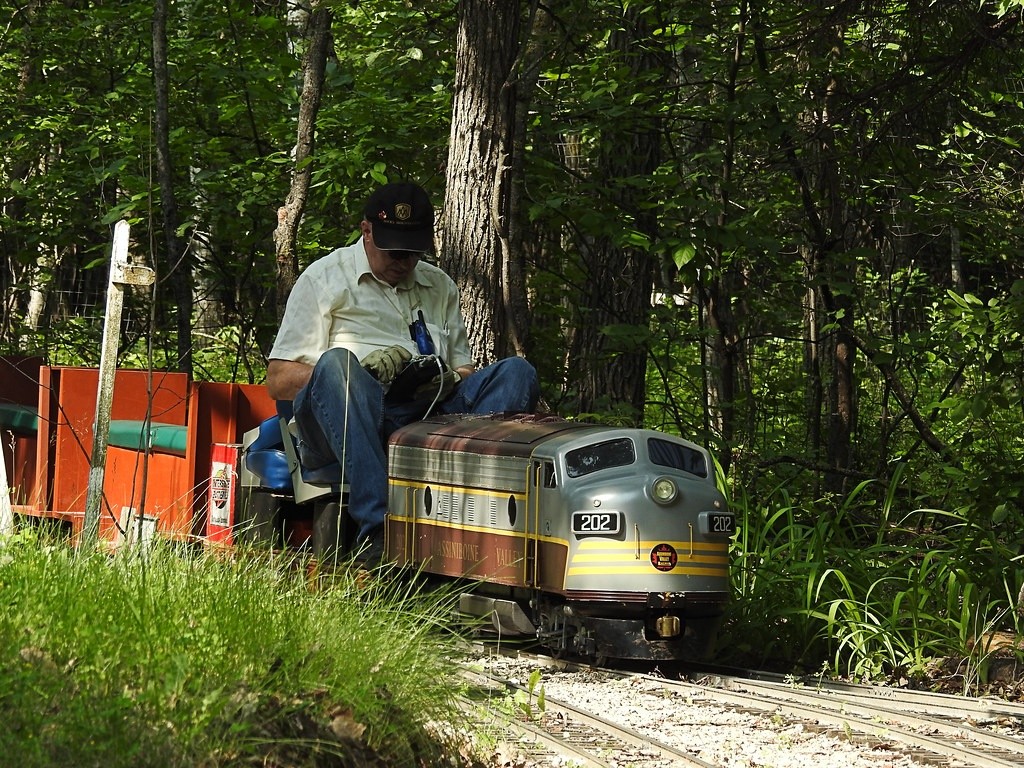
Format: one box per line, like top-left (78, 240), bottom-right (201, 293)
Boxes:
top-left (378, 251), bottom-right (413, 260)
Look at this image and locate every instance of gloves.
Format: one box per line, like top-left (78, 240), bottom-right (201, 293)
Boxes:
top-left (416, 364), bottom-right (454, 405)
top-left (358, 344), bottom-right (412, 383)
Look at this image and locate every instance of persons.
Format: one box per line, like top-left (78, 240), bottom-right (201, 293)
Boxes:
top-left (266, 182), bottom-right (539, 581)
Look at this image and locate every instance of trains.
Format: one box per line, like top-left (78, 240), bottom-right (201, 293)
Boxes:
top-left (0, 366), bottom-right (737, 671)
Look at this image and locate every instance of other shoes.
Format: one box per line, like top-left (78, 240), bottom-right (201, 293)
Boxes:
top-left (355, 528), bottom-right (384, 576)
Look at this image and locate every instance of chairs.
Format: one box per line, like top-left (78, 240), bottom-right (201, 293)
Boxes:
top-left (254, 324), bottom-right (350, 505)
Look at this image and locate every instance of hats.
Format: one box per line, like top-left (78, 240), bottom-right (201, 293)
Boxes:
top-left (364, 180), bottom-right (436, 253)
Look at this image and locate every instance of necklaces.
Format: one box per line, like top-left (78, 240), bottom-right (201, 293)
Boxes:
top-left (378, 281), bottom-right (417, 342)
